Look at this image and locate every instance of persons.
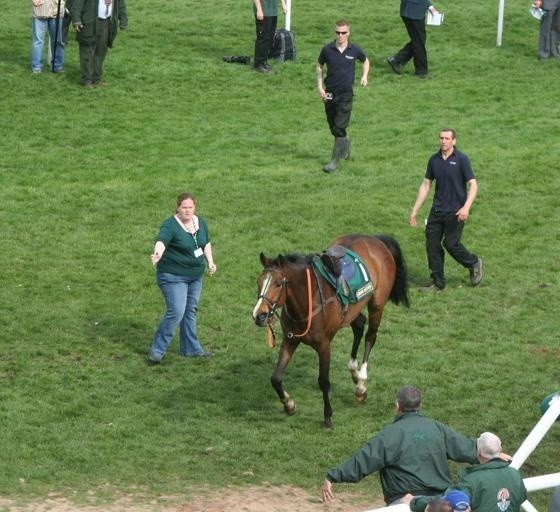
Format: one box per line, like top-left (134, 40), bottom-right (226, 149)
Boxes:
top-left (532, 1), bottom-right (559, 60)
top-left (400, 431), bottom-right (560, 512)
top-left (31, 0), bottom-right (65, 75)
top-left (252, 0), bottom-right (287, 75)
top-left (409, 129), bottom-right (483, 292)
top-left (70, 1), bottom-right (128, 86)
top-left (146, 191), bottom-right (217, 365)
top-left (59, 0), bottom-right (73, 48)
top-left (323, 386), bottom-right (514, 506)
top-left (387, 0), bottom-right (440, 80)
top-left (316, 21), bottom-right (371, 174)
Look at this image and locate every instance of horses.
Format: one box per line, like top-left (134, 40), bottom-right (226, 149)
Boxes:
top-left (251, 233), bottom-right (410, 428)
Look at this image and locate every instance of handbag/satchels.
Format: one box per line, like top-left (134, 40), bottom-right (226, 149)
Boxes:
top-left (76, 25), bottom-right (99, 44)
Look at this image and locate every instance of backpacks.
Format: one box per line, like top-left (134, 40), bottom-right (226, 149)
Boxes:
top-left (269, 28), bottom-right (298, 63)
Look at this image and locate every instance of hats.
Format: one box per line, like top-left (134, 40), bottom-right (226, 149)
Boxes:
top-left (441, 490), bottom-right (470, 510)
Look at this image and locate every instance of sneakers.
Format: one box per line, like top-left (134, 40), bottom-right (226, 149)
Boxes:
top-left (257, 64), bottom-right (272, 72)
top-left (538, 48), bottom-right (558, 59)
top-left (146, 350), bottom-right (163, 363)
top-left (193, 351), bottom-right (215, 357)
top-left (387, 57), bottom-right (429, 77)
top-left (417, 284), bottom-right (440, 292)
top-left (469, 258), bottom-right (482, 286)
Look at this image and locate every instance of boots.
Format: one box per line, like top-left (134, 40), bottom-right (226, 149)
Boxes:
top-left (323, 137), bottom-right (351, 172)
